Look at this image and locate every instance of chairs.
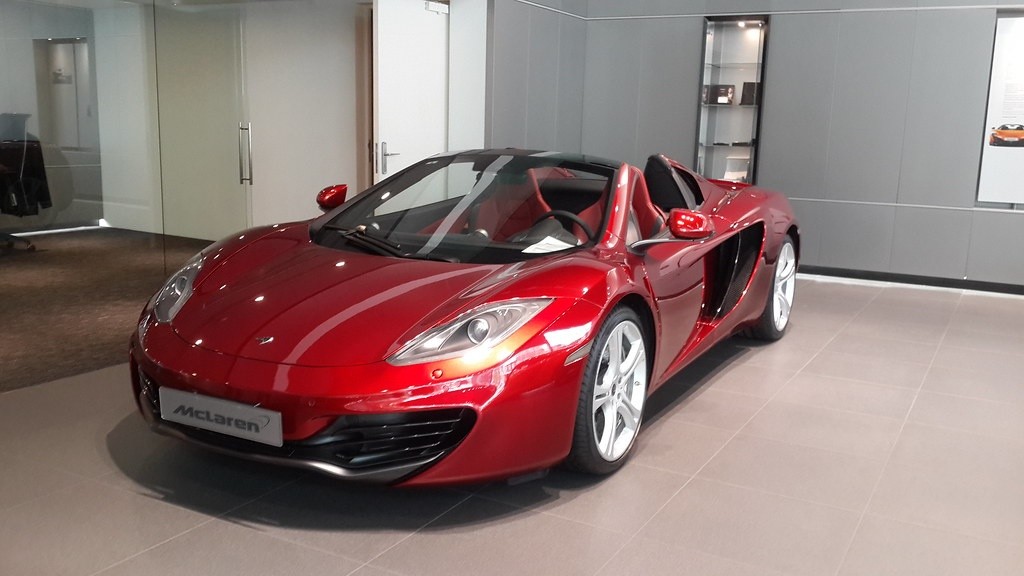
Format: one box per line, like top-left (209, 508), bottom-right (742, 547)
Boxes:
top-left (417, 166), bottom-right (555, 244)
top-left (572, 166), bottom-right (667, 248)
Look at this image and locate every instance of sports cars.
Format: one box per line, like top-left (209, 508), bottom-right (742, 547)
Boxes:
top-left (127, 147), bottom-right (802, 491)
top-left (988, 123), bottom-right (1023, 147)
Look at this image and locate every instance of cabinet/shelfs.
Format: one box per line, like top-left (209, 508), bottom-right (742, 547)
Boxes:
top-left (696, 13), bottom-right (770, 187)
